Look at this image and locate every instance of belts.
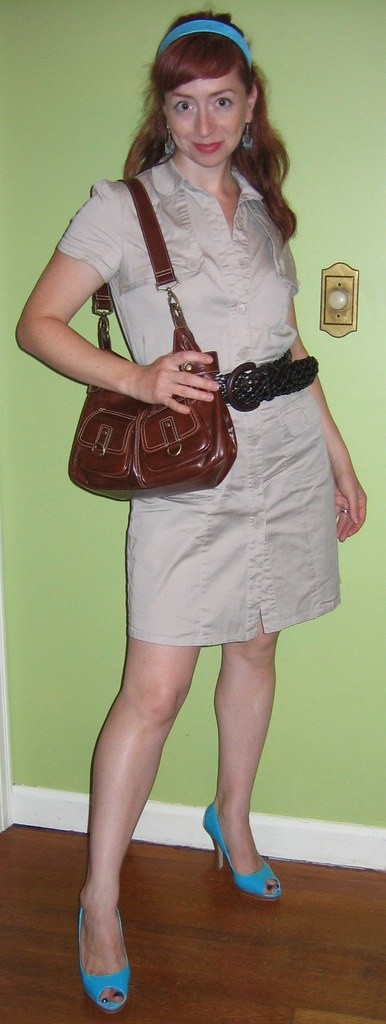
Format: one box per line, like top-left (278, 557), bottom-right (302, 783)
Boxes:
top-left (215, 348), bottom-right (319, 412)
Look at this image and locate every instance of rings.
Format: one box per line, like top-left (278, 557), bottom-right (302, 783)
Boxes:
top-left (341, 509), bottom-right (348, 514)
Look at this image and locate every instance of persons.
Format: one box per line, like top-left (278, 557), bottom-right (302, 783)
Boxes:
top-left (15, 11), bottom-right (367, 1012)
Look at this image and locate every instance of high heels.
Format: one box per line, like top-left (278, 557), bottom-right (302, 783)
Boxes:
top-left (203, 803), bottom-right (281, 901)
top-left (78, 905), bottom-right (130, 1013)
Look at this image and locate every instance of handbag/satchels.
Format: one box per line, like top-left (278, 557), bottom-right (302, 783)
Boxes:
top-left (68, 178), bottom-right (238, 501)
